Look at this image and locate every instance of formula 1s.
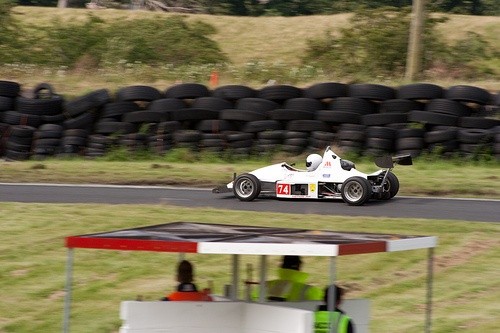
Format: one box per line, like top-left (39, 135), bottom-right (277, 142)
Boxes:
top-left (210, 146), bottom-right (413, 206)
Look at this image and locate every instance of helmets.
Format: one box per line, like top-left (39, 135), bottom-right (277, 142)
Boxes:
top-left (306, 154), bottom-right (322, 171)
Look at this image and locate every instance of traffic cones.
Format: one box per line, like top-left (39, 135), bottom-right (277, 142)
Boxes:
top-left (210, 72), bottom-right (218, 88)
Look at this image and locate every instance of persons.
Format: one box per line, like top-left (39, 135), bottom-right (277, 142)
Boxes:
top-left (306, 154), bottom-right (322, 172)
top-left (313, 284), bottom-right (354, 333)
top-left (249, 256), bottom-right (325, 302)
top-left (161, 260), bottom-right (212, 301)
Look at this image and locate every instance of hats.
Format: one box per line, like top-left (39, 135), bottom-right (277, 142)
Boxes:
top-left (325, 287), bottom-right (343, 298)
top-left (282, 256), bottom-right (301, 267)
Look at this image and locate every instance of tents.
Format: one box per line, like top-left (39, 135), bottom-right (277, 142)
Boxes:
top-left (61, 219), bottom-right (438, 333)
